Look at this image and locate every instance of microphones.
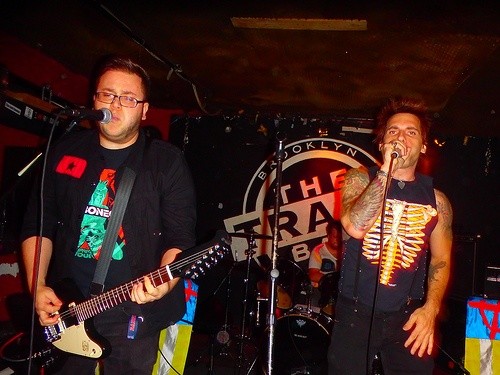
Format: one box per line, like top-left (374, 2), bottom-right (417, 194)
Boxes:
top-left (62, 108), bottom-right (112, 124)
top-left (391, 148), bottom-right (402, 158)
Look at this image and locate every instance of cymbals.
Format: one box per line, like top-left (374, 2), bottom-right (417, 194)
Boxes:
top-left (227, 230), bottom-right (274, 240)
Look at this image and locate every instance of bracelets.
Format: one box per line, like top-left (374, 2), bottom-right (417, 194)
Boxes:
top-left (376, 170), bottom-right (392, 179)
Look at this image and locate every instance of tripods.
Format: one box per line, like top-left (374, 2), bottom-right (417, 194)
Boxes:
top-left (192, 236), bottom-right (261, 375)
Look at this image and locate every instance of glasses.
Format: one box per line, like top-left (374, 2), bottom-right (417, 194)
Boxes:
top-left (93, 92), bottom-right (146, 108)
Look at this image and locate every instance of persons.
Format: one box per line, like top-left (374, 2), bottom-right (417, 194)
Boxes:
top-left (326, 99), bottom-right (453, 374)
top-left (307, 220), bottom-right (342, 311)
top-left (17, 55), bottom-right (197, 375)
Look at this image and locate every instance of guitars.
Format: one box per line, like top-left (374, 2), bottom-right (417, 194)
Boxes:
top-left (0, 228), bottom-right (233, 375)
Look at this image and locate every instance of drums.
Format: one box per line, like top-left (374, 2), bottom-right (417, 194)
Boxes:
top-left (317, 270), bottom-right (340, 307)
top-left (257, 257), bottom-right (309, 310)
top-left (263, 311), bottom-right (333, 375)
